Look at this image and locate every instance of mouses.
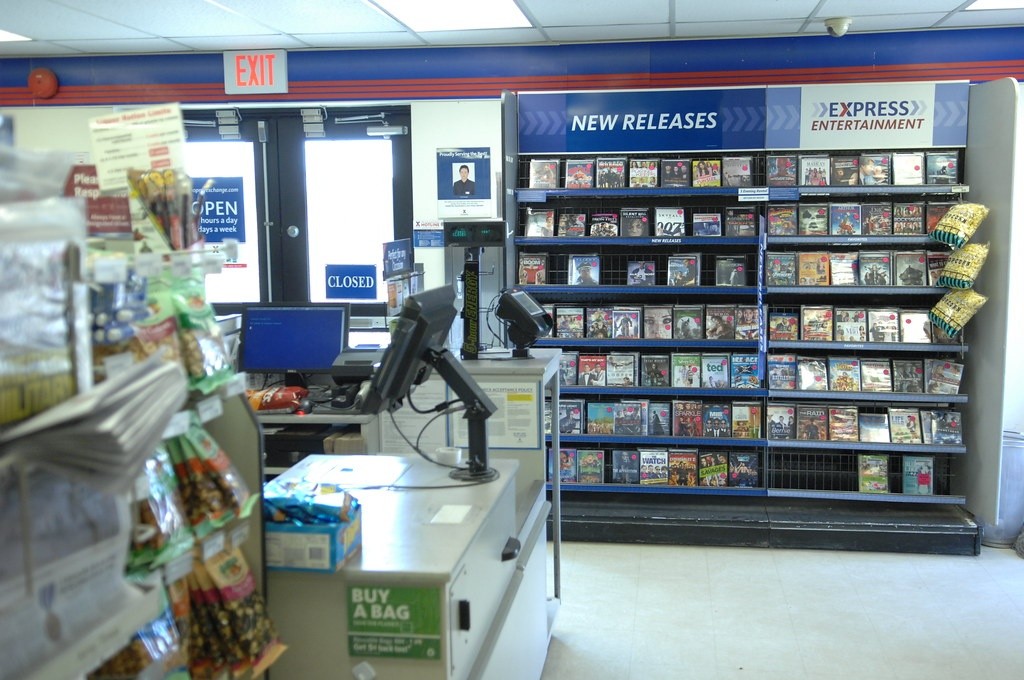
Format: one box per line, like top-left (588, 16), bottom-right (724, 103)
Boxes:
top-left (298, 399), bottom-right (313, 415)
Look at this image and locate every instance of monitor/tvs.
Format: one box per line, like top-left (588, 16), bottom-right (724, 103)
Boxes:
top-left (496, 287), bottom-right (554, 337)
top-left (358, 282), bottom-right (500, 473)
top-left (237, 302), bottom-right (351, 388)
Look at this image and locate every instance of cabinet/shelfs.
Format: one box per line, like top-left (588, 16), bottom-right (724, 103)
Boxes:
top-left (508, 182), bottom-right (970, 508)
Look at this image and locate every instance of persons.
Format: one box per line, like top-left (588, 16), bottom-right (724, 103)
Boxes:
top-left (861, 205), bottom-right (921, 235)
top-left (768, 410), bottom-right (960, 444)
top-left (772, 361), bottom-right (960, 393)
top-left (800, 258), bottom-right (942, 286)
top-left (560, 451), bottom-right (758, 486)
top-left (540, 217), bottom-right (552, 237)
top-left (557, 308), bottom-right (759, 339)
top-left (698, 223), bottom-right (716, 235)
top-left (770, 157), bottom-right (886, 186)
top-left (592, 223), bottom-right (617, 237)
top-left (453, 164), bottom-right (475, 195)
top-left (776, 312), bottom-right (897, 342)
top-left (533, 161), bottom-right (720, 188)
top-left (672, 259), bottom-right (740, 286)
top-left (523, 261), bottom-right (653, 284)
top-left (559, 407), bottom-right (748, 438)
top-left (559, 357), bottom-right (725, 387)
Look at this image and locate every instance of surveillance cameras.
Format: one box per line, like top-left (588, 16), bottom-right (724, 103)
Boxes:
top-left (824, 17), bottom-right (852, 38)
